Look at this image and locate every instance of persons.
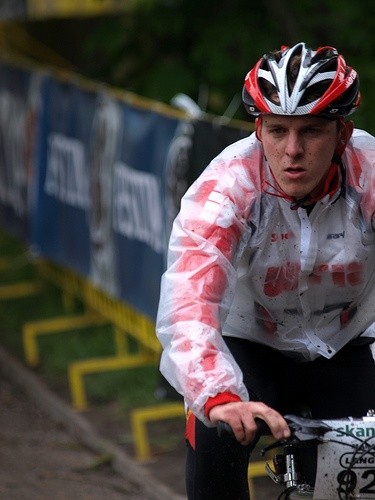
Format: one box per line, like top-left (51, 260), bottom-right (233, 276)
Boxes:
top-left (155, 42), bottom-right (375, 500)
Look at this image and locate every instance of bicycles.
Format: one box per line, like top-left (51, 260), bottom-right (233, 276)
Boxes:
top-left (222, 400), bottom-right (375, 500)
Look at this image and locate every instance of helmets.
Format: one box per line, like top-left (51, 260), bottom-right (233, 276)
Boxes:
top-left (242, 41), bottom-right (362, 120)
